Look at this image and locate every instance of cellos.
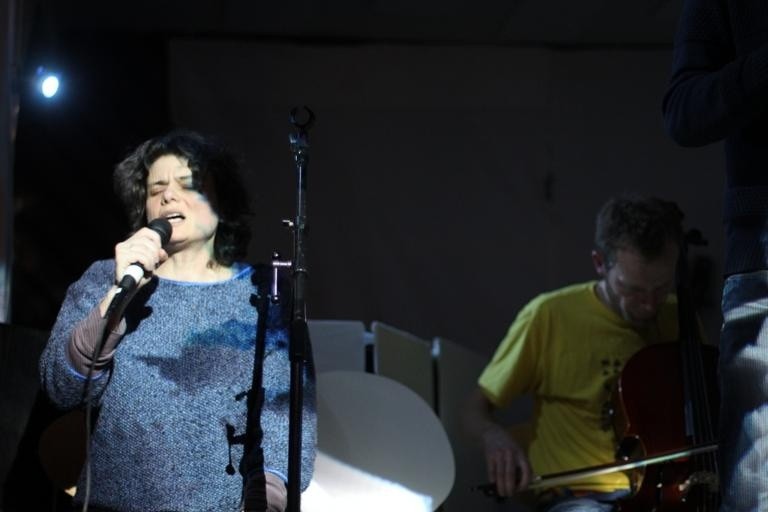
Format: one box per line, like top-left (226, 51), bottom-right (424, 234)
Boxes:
top-left (608, 202), bottom-right (718, 510)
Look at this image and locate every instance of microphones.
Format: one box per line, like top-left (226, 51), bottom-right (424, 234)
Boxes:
top-left (114, 218), bottom-right (173, 288)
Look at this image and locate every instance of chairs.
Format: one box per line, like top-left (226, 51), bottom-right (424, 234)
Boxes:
top-left (295, 371), bottom-right (456, 512)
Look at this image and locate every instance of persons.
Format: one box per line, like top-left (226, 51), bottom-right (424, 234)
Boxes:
top-left (454, 191), bottom-right (710, 512)
top-left (37, 129), bottom-right (318, 512)
